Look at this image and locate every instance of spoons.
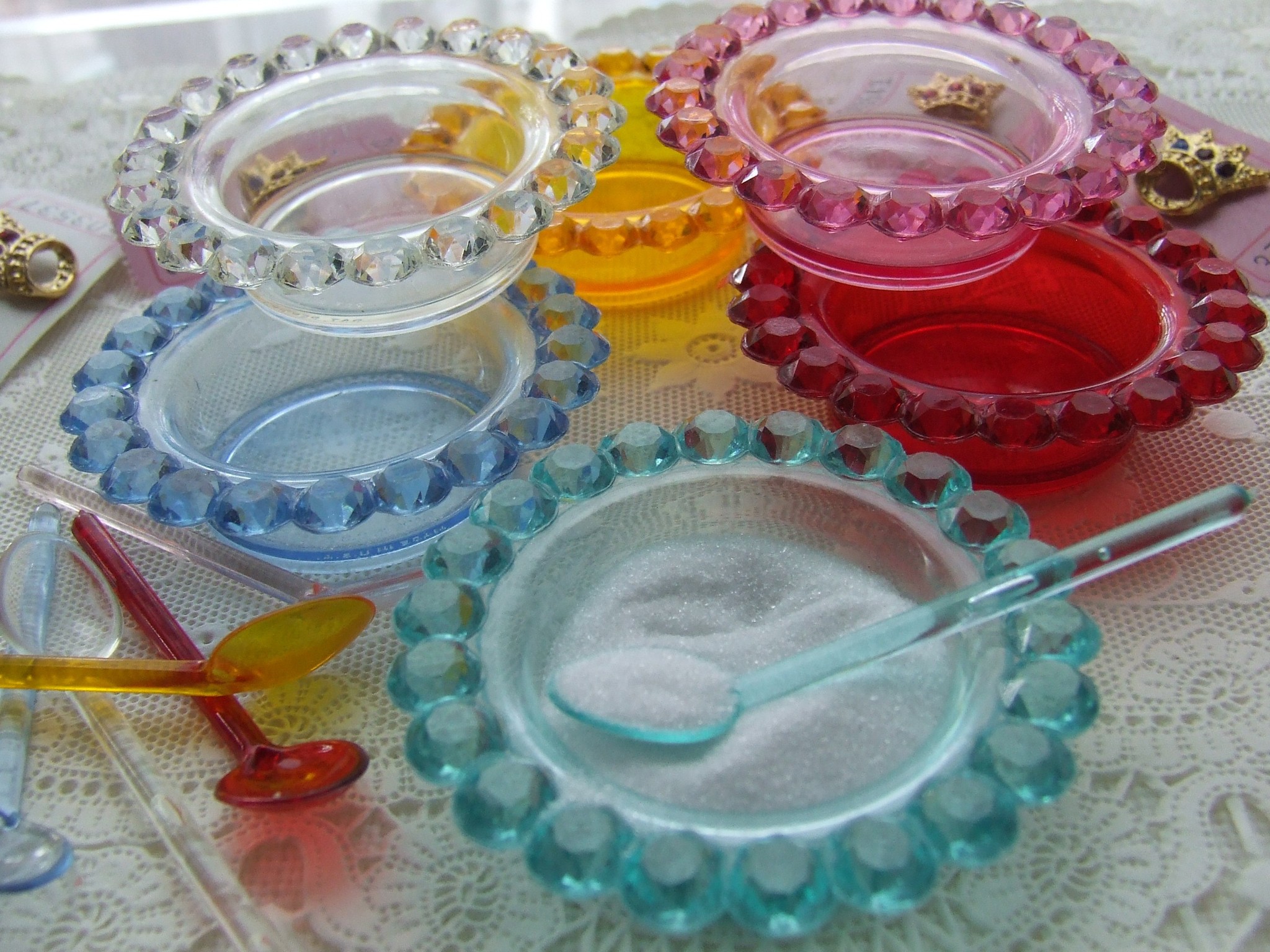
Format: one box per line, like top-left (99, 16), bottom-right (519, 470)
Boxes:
top-left (0, 536), bottom-right (292, 952)
top-left (541, 480), bottom-right (1252, 746)
top-left (0, 499), bottom-right (75, 894)
top-left (1, 595), bottom-right (378, 699)
top-left (70, 508), bottom-right (373, 809)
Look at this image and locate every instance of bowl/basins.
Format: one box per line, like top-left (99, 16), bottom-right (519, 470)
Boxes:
top-left (641, 0), bottom-right (1169, 294)
top-left (722, 202), bottom-right (1269, 486)
top-left (101, 16), bottom-right (628, 340)
top-left (387, 410), bottom-right (1103, 940)
top-left (396, 47), bottom-right (831, 312)
top-left (56, 259), bottom-right (610, 575)
